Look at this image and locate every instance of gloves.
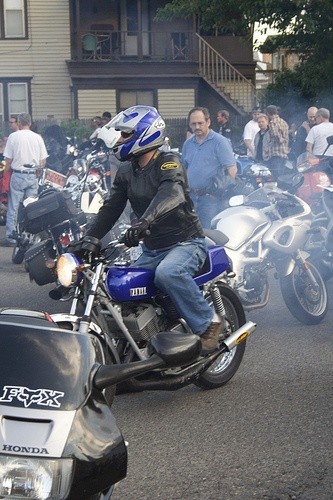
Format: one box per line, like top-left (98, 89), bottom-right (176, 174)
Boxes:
top-left (124, 219), bottom-right (149, 247)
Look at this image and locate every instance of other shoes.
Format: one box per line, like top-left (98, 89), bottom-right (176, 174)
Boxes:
top-left (0, 239), bottom-right (17, 247)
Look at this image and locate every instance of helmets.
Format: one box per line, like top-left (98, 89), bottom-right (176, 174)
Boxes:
top-left (105, 105), bottom-right (166, 162)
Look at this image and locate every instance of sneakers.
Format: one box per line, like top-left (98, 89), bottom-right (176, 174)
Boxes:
top-left (200, 316), bottom-right (225, 355)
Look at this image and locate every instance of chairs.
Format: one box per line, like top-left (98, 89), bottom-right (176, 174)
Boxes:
top-left (90, 24), bottom-right (117, 52)
top-left (169, 32), bottom-right (189, 61)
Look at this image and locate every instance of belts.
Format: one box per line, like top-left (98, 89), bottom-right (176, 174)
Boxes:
top-left (190, 189), bottom-right (212, 197)
top-left (13, 170), bottom-right (36, 174)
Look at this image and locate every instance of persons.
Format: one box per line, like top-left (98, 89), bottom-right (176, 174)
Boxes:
top-left (8, 114), bottom-right (67, 175)
top-left (91, 111), bottom-right (111, 131)
top-left (80, 105), bottom-right (226, 352)
top-left (0, 113), bottom-right (50, 247)
top-left (217, 109), bottom-right (232, 142)
top-left (187, 119), bottom-right (193, 139)
top-left (181, 107), bottom-right (236, 230)
top-left (242, 105), bottom-right (333, 187)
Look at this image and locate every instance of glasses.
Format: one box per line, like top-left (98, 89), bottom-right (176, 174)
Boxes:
top-left (120, 131), bottom-right (133, 138)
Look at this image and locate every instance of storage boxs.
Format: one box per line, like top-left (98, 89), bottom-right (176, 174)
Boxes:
top-left (21, 190), bottom-right (77, 234)
top-left (24, 239), bottom-right (57, 286)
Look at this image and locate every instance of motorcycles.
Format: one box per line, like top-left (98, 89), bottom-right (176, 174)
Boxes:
top-left (195, 156), bottom-right (328, 326)
top-left (0, 137), bottom-right (333, 314)
top-left (44, 218), bottom-right (258, 413)
top-left (0, 302), bottom-right (202, 500)
